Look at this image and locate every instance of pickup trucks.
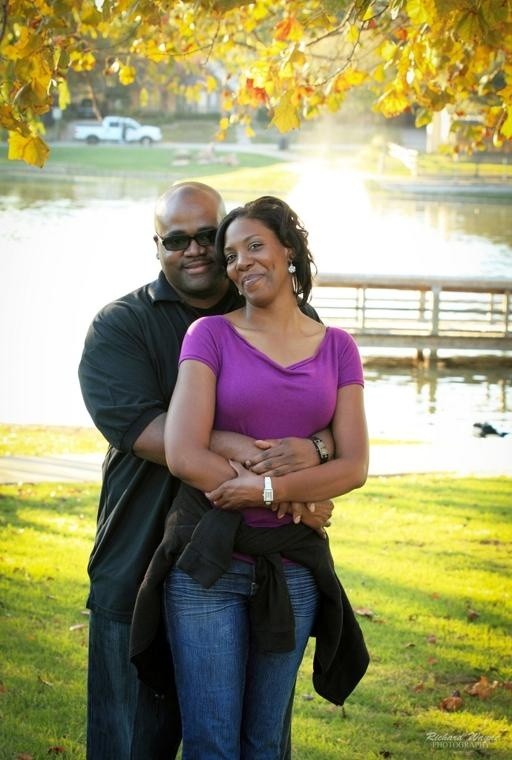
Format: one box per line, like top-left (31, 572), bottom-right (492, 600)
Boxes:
top-left (72, 114), bottom-right (164, 147)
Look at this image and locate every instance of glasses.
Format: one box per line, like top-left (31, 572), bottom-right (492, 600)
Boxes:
top-left (151, 228), bottom-right (218, 251)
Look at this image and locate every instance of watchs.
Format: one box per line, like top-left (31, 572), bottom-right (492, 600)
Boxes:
top-left (309, 434), bottom-right (330, 465)
top-left (263, 475), bottom-right (273, 511)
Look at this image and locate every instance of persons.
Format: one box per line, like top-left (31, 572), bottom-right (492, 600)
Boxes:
top-left (78, 180), bottom-right (349, 759)
top-left (162, 196), bottom-right (371, 760)
